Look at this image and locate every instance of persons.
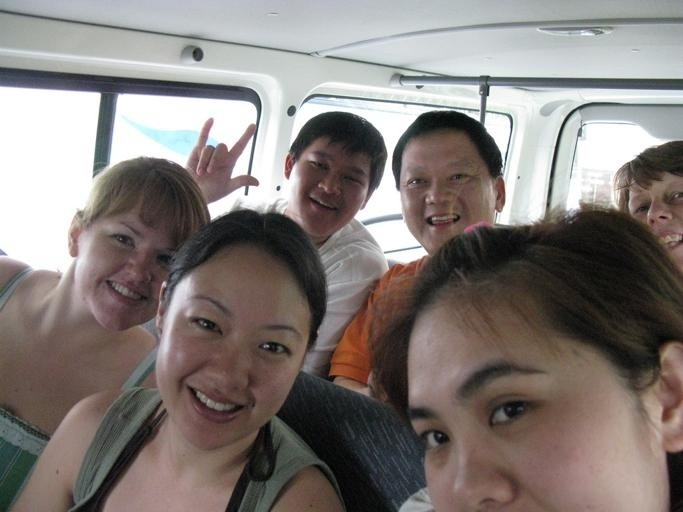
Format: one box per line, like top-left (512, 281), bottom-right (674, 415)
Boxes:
top-left (330, 112), bottom-right (506, 407)
top-left (0, 157), bottom-right (210, 512)
top-left (7, 211), bottom-right (346, 512)
top-left (187, 112), bottom-right (386, 377)
top-left (368, 209), bottom-right (682, 510)
top-left (613, 141), bottom-right (683, 274)
